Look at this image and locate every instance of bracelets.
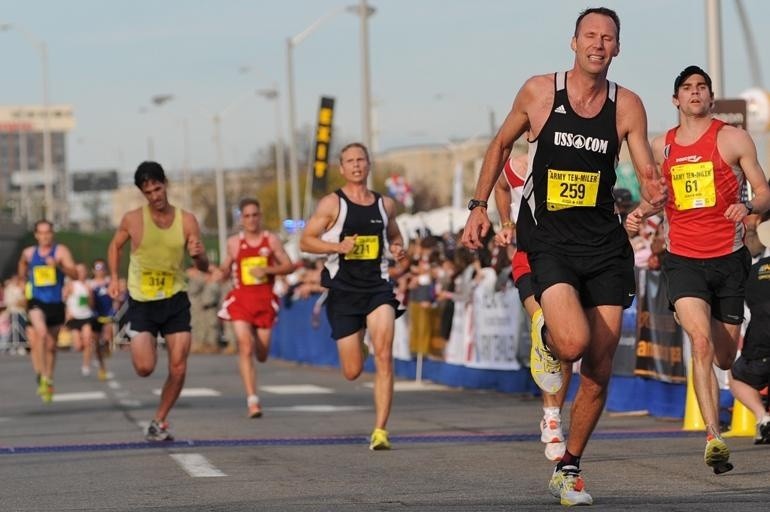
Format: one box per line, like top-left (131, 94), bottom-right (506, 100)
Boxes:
top-left (503, 223), bottom-right (515, 228)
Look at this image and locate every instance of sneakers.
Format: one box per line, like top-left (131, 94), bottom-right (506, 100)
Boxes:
top-left (705, 434), bottom-right (735, 475)
top-left (100, 371), bottom-right (106, 381)
top-left (545, 440), bottom-right (568, 461)
top-left (753, 417), bottom-right (769, 445)
top-left (368, 428), bottom-right (392, 450)
top-left (41, 381), bottom-right (53, 403)
top-left (249, 404), bottom-right (261, 418)
top-left (549, 467), bottom-right (593, 505)
top-left (82, 364), bottom-right (90, 375)
top-left (361, 343), bottom-right (369, 364)
top-left (146, 420), bottom-right (173, 442)
top-left (540, 413), bottom-right (565, 443)
top-left (36, 378), bottom-right (47, 394)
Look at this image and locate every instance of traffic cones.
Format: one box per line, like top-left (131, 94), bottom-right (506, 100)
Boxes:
top-left (680, 357), bottom-right (707, 431)
top-left (719, 398), bottom-right (761, 439)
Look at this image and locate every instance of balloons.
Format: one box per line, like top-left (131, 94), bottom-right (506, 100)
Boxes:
top-left (384, 175), bottom-right (416, 208)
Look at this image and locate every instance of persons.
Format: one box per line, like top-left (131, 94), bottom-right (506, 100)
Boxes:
top-left (613, 185), bottom-right (668, 269)
top-left (2, 274), bottom-right (31, 355)
top-left (627, 66), bottom-right (769, 475)
top-left (213, 197), bottom-right (294, 417)
top-left (295, 144), bottom-right (408, 450)
top-left (184, 263), bottom-right (235, 354)
top-left (107, 161), bottom-right (208, 442)
top-left (496, 131), bottom-right (572, 463)
top-left (62, 263), bottom-right (94, 378)
top-left (408, 223), bottom-right (462, 303)
top-left (19, 221), bottom-right (78, 405)
top-left (91, 259), bottom-right (114, 381)
top-left (728, 211), bottom-right (770, 445)
top-left (462, 8), bottom-right (668, 505)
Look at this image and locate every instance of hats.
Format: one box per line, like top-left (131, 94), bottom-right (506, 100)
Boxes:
top-left (613, 187), bottom-right (632, 204)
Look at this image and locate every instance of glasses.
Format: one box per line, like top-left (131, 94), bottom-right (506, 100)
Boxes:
top-left (622, 203), bottom-right (635, 210)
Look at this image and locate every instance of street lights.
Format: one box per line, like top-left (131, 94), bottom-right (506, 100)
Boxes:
top-left (285, 4), bottom-right (377, 237)
top-left (154, 91), bottom-right (228, 267)
top-left (0, 21), bottom-right (55, 222)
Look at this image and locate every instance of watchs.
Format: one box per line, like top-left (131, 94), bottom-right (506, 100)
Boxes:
top-left (741, 200), bottom-right (753, 216)
top-left (468, 200), bottom-right (487, 210)
